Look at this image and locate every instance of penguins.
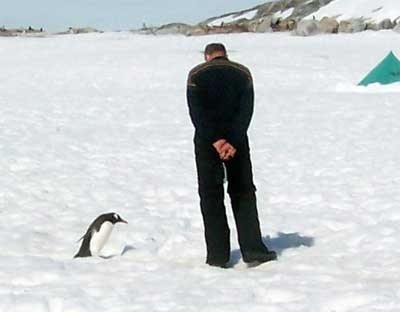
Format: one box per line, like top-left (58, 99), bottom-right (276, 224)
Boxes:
top-left (72, 211), bottom-right (128, 259)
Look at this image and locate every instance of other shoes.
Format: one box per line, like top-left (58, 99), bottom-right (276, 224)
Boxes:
top-left (244, 250), bottom-right (276, 263)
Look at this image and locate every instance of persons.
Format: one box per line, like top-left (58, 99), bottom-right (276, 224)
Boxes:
top-left (187, 43), bottom-right (276, 267)
